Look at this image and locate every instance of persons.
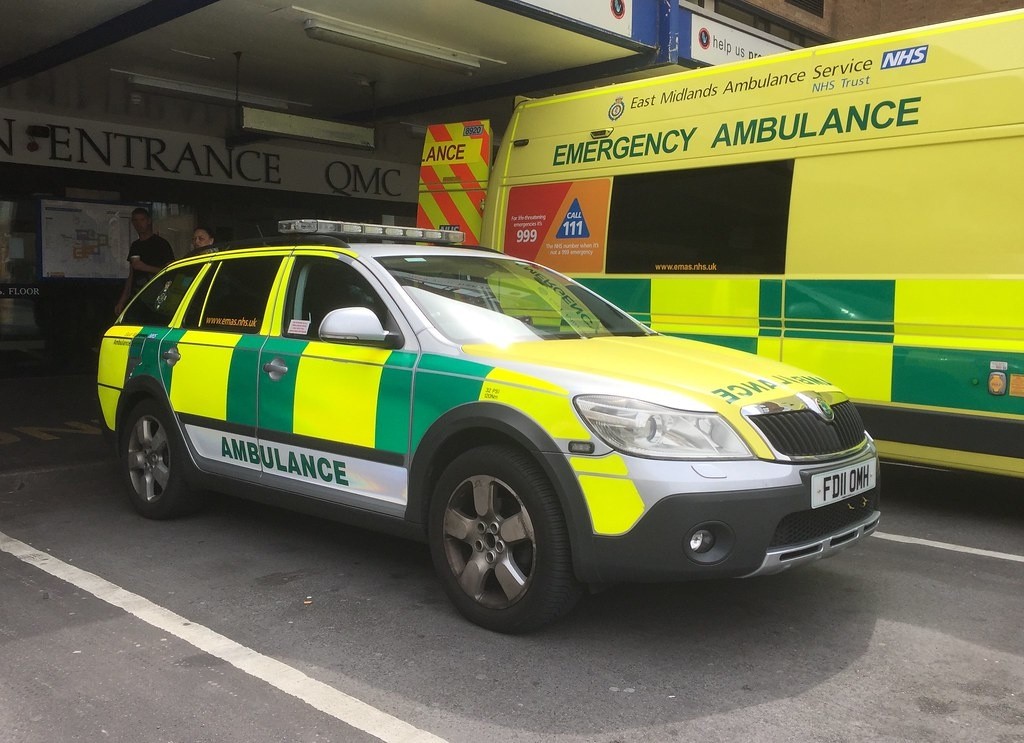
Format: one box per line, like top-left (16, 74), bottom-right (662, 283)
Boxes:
top-left (113, 208), bottom-right (175, 316)
top-left (177, 226), bottom-right (220, 279)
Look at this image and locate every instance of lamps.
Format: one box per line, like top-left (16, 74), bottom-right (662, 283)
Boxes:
top-left (301, 19), bottom-right (481, 76)
top-left (123, 76), bottom-right (288, 113)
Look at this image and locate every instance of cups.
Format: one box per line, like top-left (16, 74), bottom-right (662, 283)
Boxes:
top-left (130, 255), bottom-right (140, 268)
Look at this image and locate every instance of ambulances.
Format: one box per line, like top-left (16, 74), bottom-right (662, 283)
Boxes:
top-left (94, 215), bottom-right (884, 638)
top-left (414, 8), bottom-right (1024, 508)
